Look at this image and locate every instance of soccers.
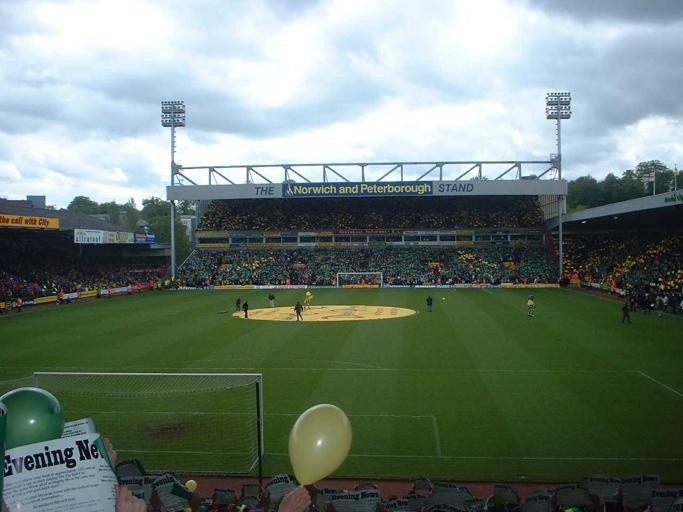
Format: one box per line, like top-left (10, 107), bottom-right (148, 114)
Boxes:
top-left (442, 297), bottom-right (445, 300)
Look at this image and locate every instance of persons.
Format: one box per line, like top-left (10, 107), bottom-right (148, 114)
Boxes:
top-left (0, 245), bottom-right (170, 314)
top-left (99, 436), bottom-right (148, 512)
top-left (174, 199), bottom-right (559, 286)
top-left (235, 295), bottom-right (241, 310)
top-left (242, 300), bottom-right (248, 320)
top-left (526, 294), bottom-right (536, 317)
top-left (268, 292), bottom-right (275, 307)
top-left (277, 483), bottom-right (313, 512)
top-left (293, 300), bottom-right (303, 321)
top-left (621, 301), bottom-right (631, 324)
top-left (561, 223), bottom-right (682, 317)
top-left (304, 290), bottom-right (313, 309)
top-left (426, 295), bottom-right (433, 312)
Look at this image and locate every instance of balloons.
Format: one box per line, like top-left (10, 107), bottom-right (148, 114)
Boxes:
top-left (0, 386), bottom-right (65, 449)
top-left (284, 402), bottom-right (352, 487)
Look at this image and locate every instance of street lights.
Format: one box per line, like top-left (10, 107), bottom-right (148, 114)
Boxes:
top-left (545, 92), bottom-right (572, 284)
top-left (160, 100), bottom-right (186, 280)
